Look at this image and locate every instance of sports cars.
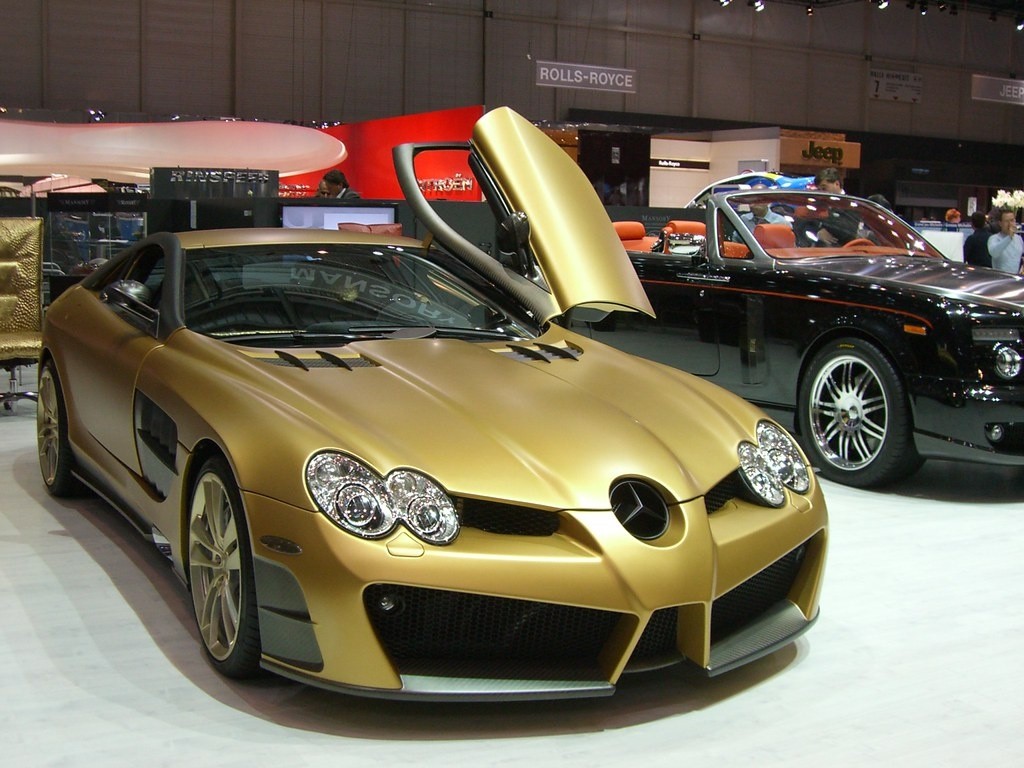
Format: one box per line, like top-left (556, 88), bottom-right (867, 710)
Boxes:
top-left (36, 106), bottom-right (830, 704)
top-left (557, 189), bottom-right (1024, 492)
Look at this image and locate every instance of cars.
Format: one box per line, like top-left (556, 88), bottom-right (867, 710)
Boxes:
top-left (685, 171), bottom-right (817, 209)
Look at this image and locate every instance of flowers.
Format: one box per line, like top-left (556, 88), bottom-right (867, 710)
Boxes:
top-left (992, 190), bottom-right (1024, 218)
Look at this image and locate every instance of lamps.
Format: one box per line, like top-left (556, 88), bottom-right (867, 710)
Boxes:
top-left (920, 4), bottom-right (929, 16)
top-left (806, 6), bottom-right (814, 15)
top-left (870, 0), bottom-right (888, 10)
top-left (748, 0), bottom-right (765, 13)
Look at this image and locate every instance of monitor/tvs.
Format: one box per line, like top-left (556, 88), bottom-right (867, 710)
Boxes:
top-left (279, 202), bottom-right (399, 231)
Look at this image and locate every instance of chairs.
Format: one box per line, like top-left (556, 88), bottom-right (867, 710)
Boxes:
top-left (752, 221), bottom-right (800, 256)
top-left (0, 216), bottom-right (46, 415)
top-left (613, 222), bottom-right (659, 250)
top-left (662, 219), bottom-right (714, 254)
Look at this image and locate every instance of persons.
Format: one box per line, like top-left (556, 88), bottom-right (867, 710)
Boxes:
top-left (985, 207), bottom-right (1001, 233)
top-left (732, 184), bottom-right (793, 244)
top-left (964, 212), bottom-right (995, 268)
top-left (945, 209), bottom-right (961, 232)
top-left (316, 180), bottom-right (334, 198)
top-left (798, 168), bottom-right (913, 246)
top-left (987, 210), bottom-right (1024, 276)
top-left (323, 169), bottom-right (360, 199)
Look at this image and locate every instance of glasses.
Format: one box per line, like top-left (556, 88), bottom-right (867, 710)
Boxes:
top-left (319, 190), bottom-right (330, 195)
